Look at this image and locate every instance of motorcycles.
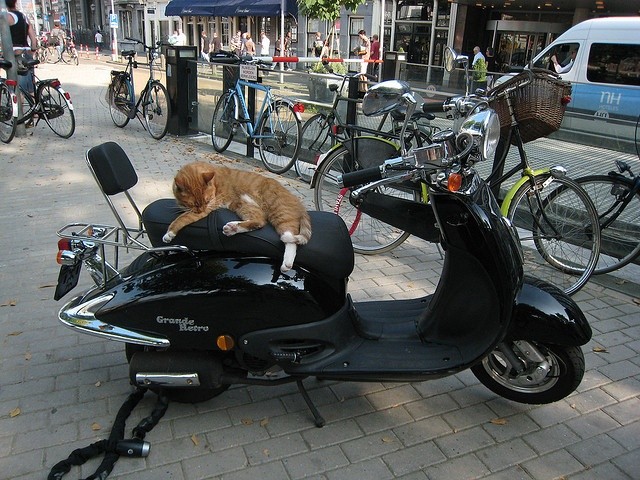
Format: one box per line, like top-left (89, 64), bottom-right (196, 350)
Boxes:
top-left (53, 47), bottom-right (592, 427)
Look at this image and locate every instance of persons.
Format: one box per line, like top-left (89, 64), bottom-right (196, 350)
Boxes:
top-left (171, 28), bottom-right (187, 46)
top-left (471, 45), bottom-right (487, 93)
top-left (51, 22), bottom-right (67, 62)
top-left (94, 30), bottom-right (103, 51)
top-left (312, 31), bottom-right (323, 57)
top-left (230, 30), bottom-right (241, 55)
top-left (211, 32), bottom-right (223, 52)
top-left (245, 33), bottom-right (255, 57)
top-left (370, 34), bottom-right (380, 77)
top-left (320, 39), bottom-right (329, 65)
top-left (258, 32), bottom-right (270, 56)
top-left (352, 30), bottom-right (371, 92)
top-left (484, 46), bottom-right (497, 71)
top-left (240, 33), bottom-right (248, 55)
top-left (551, 46), bottom-right (580, 75)
top-left (5, 0), bottom-right (38, 126)
top-left (200, 30), bottom-right (211, 71)
top-left (270, 34), bottom-right (281, 69)
top-left (284, 31), bottom-right (292, 71)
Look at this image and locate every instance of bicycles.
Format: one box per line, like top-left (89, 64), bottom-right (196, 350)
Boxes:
top-left (212, 49), bottom-right (305, 174)
top-left (309, 65), bottom-right (601, 296)
top-left (0, 60), bottom-right (19, 144)
top-left (61, 38), bottom-right (78, 64)
top-left (33, 45), bottom-right (61, 64)
top-left (294, 57), bottom-right (440, 184)
top-left (533, 159), bottom-right (639, 276)
top-left (109, 37), bottom-right (171, 139)
top-left (0, 58), bottom-right (75, 140)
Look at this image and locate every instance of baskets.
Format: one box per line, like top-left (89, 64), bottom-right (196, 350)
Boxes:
top-left (488, 71), bottom-right (573, 147)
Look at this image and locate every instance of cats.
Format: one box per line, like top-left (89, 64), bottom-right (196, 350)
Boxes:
top-left (161, 159), bottom-right (312, 272)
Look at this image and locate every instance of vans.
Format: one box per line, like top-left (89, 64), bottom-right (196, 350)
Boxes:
top-left (494, 16), bottom-right (640, 145)
